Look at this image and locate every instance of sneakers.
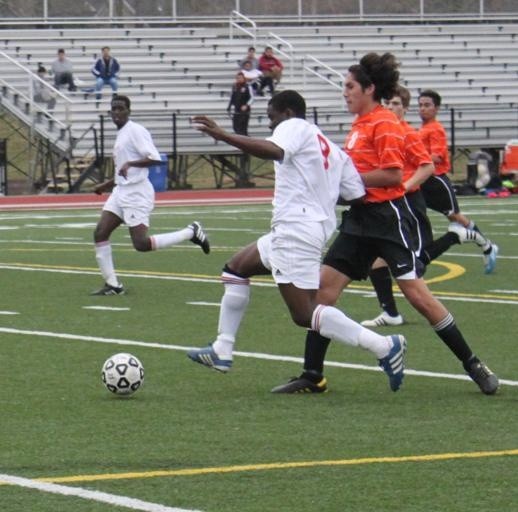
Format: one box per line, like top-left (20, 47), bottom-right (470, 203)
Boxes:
top-left (464, 357), bottom-right (498, 395)
top-left (187, 346), bottom-right (235, 372)
top-left (447, 226), bottom-right (483, 246)
top-left (482, 240), bottom-right (499, 277)
top-left (359, 313), bottom-right (405, 329)
top-left (377, 335), bottom-right (406, 394)
top-left (186, 219), bottom-right (209, 256)
top-left (270, 373), bottom-right (329, 396)
top-left (89, 282), bottom-right (128, 298)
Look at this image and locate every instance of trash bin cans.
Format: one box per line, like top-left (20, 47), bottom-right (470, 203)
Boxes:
top-left (148, 154), bottom-right (169, 193)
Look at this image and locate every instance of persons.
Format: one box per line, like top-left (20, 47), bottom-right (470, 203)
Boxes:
top-left (269, 52), bottom-right (498, 396)
top-left (186, 89), bottom-right (406, 393)
top-left (48, 48), bottom-right (77, 93)
top-left (361, 83), bottom-right (486, 328)
top-left (91, 96), bottom-right (210, 296)
top-left (414, 89), bottom-right (499, 275)
top-left (227, 45), bottom-right (284, 137)
top-left (91, 46), bottom-right (120, 100)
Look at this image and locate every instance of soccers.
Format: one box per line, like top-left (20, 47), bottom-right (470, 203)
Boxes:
top-left (101, 354), bottom-right (145, 396)
